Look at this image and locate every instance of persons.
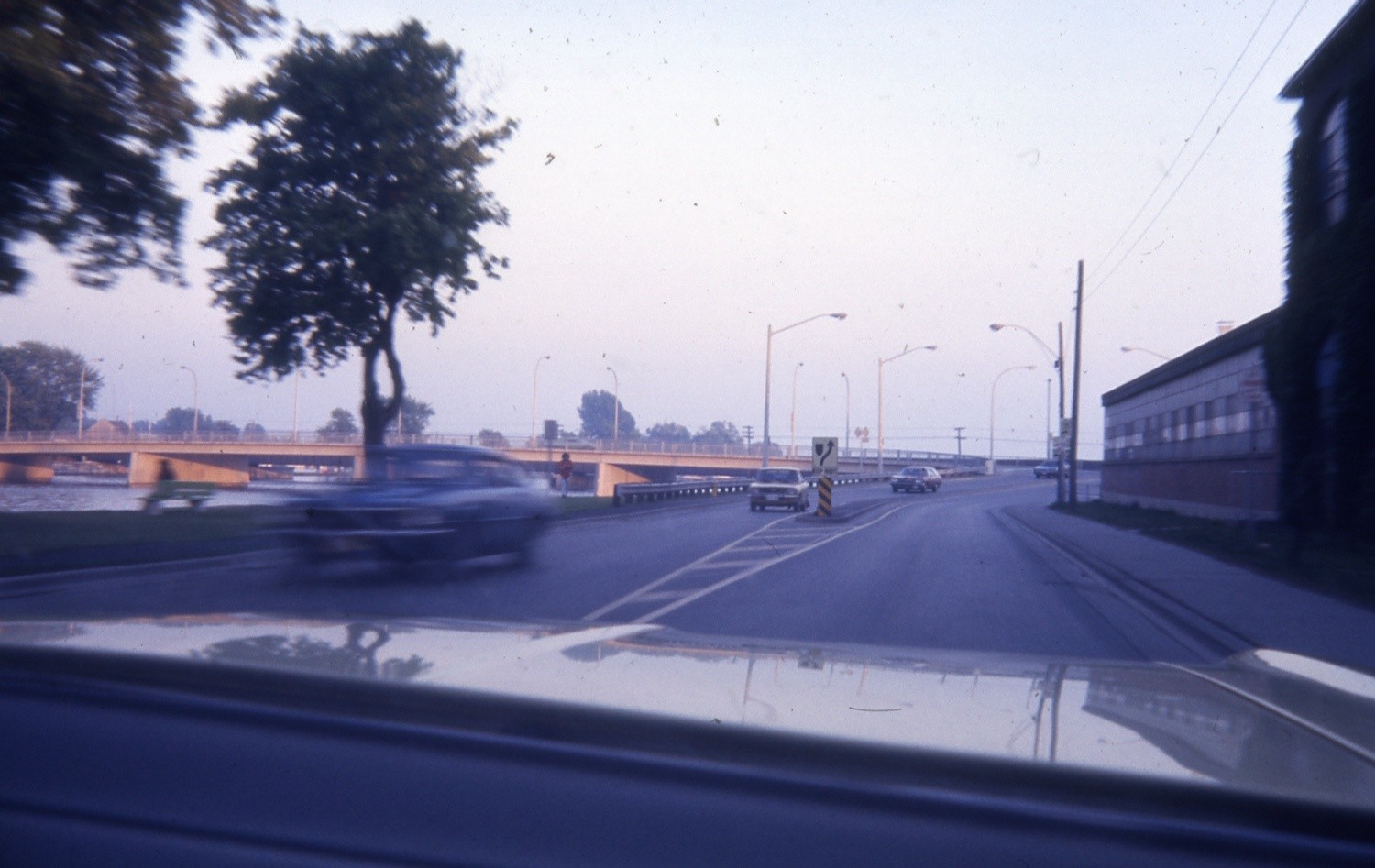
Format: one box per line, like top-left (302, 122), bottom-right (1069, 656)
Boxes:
top-left (558, 451), bottom-right (574, 497)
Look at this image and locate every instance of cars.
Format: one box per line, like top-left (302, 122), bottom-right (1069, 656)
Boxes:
top-left (283, 447), bottom-right (563, 582)
top-left (747, 467), bottom-right (812, 513)
top-left (1034, 460), bottom-right (1070, 479)
top-left (891, 466), bottom-right (942, 493)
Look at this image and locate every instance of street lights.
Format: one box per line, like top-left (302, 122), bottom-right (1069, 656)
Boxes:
top-left (763, 312), bottom-right (848, 468)
top-left (989, 366), bottom-right (1040, 474)
top-left (990, 322), bottom-right (1065, 502)
top-left (0, 358), bottom-right (406, 444)
top-left (531, 354), bottom-right (620, 452)
top-left (878, 344), bottom-right (938, 484)
